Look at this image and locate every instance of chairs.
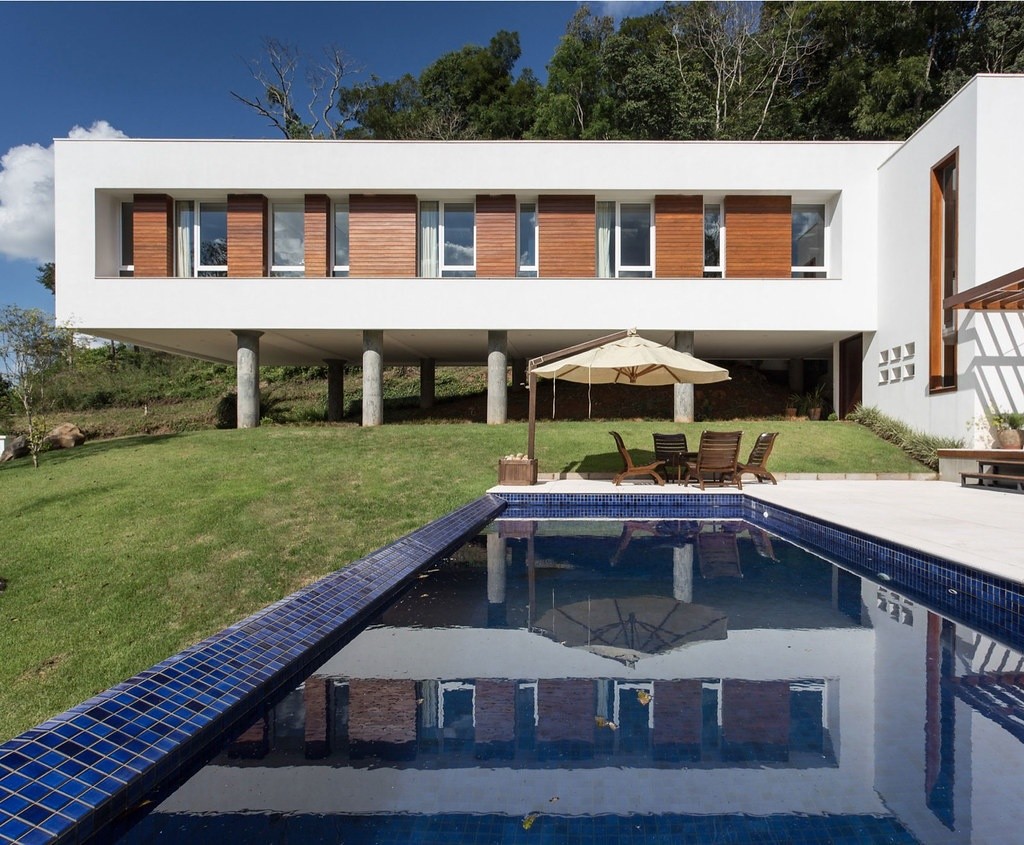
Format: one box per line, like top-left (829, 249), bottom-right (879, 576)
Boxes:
top-left (685, 430), bottom-right (744, 490)
top-left (690, 521), bottom-right (745, 578)
top-left (609, 431), bottom-right (666, 486)
top-left (652, 433), bottom-right (689, 485)
top-left (728, 432), bottom-right (780, 485)
top-left (723, 520), bottom-right (781, 564)
top-left (610, 521), bottom-right (666, 567)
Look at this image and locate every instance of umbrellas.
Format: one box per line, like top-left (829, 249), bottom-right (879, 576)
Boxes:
top-left (528, 329), bottom-right (732, 459)
top-left (527, 538), bottom-right (726, 669)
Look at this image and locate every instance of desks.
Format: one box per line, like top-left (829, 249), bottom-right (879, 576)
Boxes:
top-left (655, 452), bottom-right (723, 485)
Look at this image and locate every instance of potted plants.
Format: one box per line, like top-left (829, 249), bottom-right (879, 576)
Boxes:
top-left (787, 382), bottom-right (833, 420)
top-left (966, 397), bottom-right (1024, 450)
top-left (784, 402), bottom-right (797, 418)
